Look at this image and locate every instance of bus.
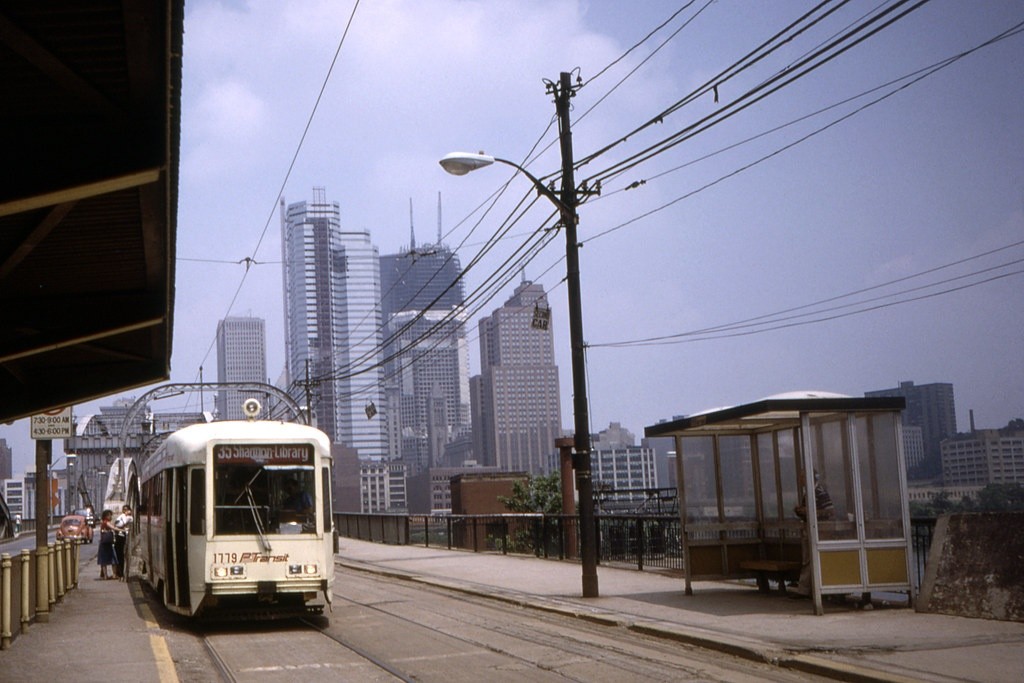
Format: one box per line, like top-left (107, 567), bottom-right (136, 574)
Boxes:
top-left (122, 397), bottom-right (339, 623)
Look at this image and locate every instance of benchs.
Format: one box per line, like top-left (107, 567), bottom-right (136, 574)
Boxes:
top-left (739, 559), bottom-right (802, 594)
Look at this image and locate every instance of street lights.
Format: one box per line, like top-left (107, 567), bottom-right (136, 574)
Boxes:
top-left (49, 453), bottom-right (76, 530)
top-left (97, 472), bottom-right (107, 517)
top-left (437, 151), bottom-right (601, 600)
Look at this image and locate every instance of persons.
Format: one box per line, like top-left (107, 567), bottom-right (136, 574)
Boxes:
top-left (115, 510), bottom-right (133, 537)
top-left (789, 469), bottom-right (836, 601)
top-left (185, 467), bottom-right (313, 534)
top-left (97, 509), bottom-right (128, 580)
top-left (113, 505), bottom-right (132, 577)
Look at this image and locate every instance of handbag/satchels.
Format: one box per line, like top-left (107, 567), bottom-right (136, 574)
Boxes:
top-left (102, 524), bottom-right (114, 544)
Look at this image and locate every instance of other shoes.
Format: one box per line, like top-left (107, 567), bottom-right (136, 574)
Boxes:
top-left (785, 586), bottom-right (808, 596)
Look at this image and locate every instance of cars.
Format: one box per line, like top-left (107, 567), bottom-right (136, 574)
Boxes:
top-left (55, 514), bottom-right (95, 544)
top-left (73, 507), bottom-right (96, 526)
